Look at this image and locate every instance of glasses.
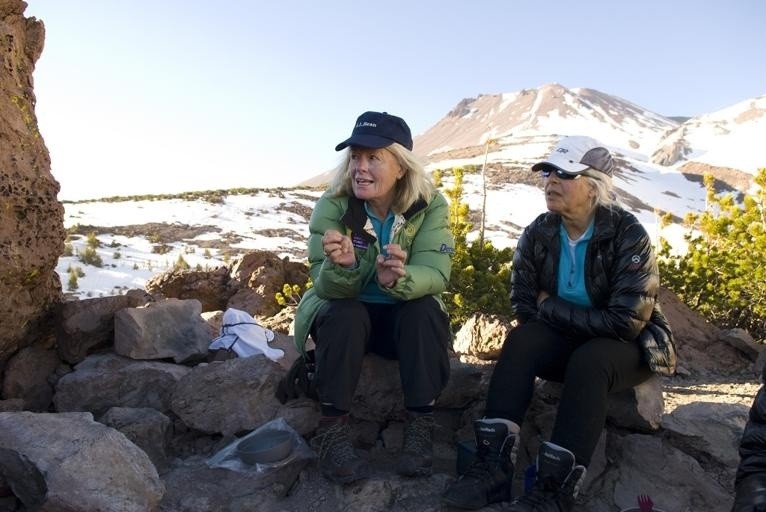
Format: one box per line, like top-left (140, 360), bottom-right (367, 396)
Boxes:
top-left (540, 167), bottom-right (601, 180)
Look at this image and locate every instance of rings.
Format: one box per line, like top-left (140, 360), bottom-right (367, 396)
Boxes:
top-left (322, 250), bottom-right (331, 256)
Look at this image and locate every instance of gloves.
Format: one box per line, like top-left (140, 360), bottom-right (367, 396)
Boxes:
top-left (277, 350), bottom-right (314, 405)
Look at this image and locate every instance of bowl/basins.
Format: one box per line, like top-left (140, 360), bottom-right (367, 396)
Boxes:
top-left (236, 429), bottom-right (293, 465)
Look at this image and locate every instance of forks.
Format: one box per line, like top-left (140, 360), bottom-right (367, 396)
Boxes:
top-left (636, 493), bottom-right (653, 512)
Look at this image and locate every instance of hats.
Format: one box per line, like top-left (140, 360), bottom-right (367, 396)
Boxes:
top-left (531, 136), bottom-right (612, 178)
top-left (335, 111), bottom-right (412, 151)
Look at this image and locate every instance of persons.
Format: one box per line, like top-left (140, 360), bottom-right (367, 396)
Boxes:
top-left (442, 135), bottom-right (668, 512)
top-left (731, 382), bottom-right (766, 512)
top-left (300, 111), bottom-right (456, 485)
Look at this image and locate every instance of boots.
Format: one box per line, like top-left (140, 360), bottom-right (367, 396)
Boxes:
top-left (501, 442), bottom-right (586, 512)
top-left (400, 411), bottom-right (434, 478)
top-left (316, 416), bottom-right (352, 484)
top-left (442, 419), bottom-right (520, 509)
